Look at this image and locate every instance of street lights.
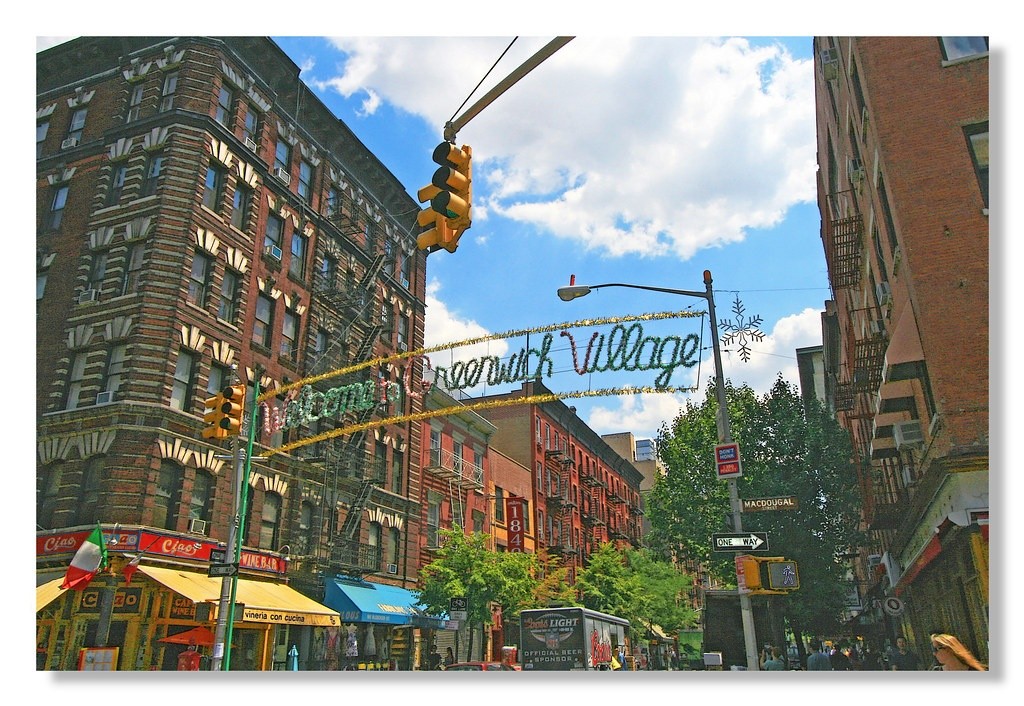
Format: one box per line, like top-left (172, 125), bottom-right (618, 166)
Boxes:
top-left (557, 270), bottom-right (760, 672)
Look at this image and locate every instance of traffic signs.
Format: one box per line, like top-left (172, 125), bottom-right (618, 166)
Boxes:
top-left (210, 547), bottom-right (236, 562)
top-left (711, 532), bottom-right (770, 552)
top-left (208, 562), bottom-right (239, 578)
top-left (768, 561), bottom-right (799, 590)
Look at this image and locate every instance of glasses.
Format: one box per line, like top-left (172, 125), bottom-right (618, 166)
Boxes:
top-left (930, 644), bottom-right (945, 652)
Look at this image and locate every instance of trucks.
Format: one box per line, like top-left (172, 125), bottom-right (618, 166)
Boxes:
top-left (519, 606), bottom-right (630, 671)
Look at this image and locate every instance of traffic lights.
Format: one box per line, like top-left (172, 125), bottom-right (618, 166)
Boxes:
top-left (203, 392), bottom-right (227, 439)
top-left (432, 140), bottom-right (472, 230)
top-left (220, 384), bottom-right (244, 435)
top-left (417, 182), bottom-right (453, 251)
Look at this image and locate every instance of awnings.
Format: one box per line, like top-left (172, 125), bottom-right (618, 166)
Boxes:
top-left (37, 568), bottom-right (101, 612)
top-left (324, 578), bottom-right (450, 630)
top-left (869, 299), bottom-right (924, 460)
top-left (137, 565), bottom-right (341, 626)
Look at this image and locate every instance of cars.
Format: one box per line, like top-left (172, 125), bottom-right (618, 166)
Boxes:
top-left (445, 661), bottom-right (516, 672)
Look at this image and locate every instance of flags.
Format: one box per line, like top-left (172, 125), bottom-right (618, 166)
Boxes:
top-left (61, 525), bottom-right (107, 592)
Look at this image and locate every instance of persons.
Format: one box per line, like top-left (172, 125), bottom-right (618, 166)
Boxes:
top-left (663, 650), bottom-right (678, 670)
top-left (312, 623), bottom-right (388, 671)
top-left (420, 645), bottom-right (454, 670)
top-left (760, 631), bottom-right (921, 671)
top-left (614, 648), bottom-right (628, 671)
top-left (930, 634), bottom-right (989, 671)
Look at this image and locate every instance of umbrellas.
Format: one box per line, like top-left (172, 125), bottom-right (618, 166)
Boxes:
top-left (288, 645), bottom-right (298, 671)
top-left (157, 625), bottom-right (237, 652)
